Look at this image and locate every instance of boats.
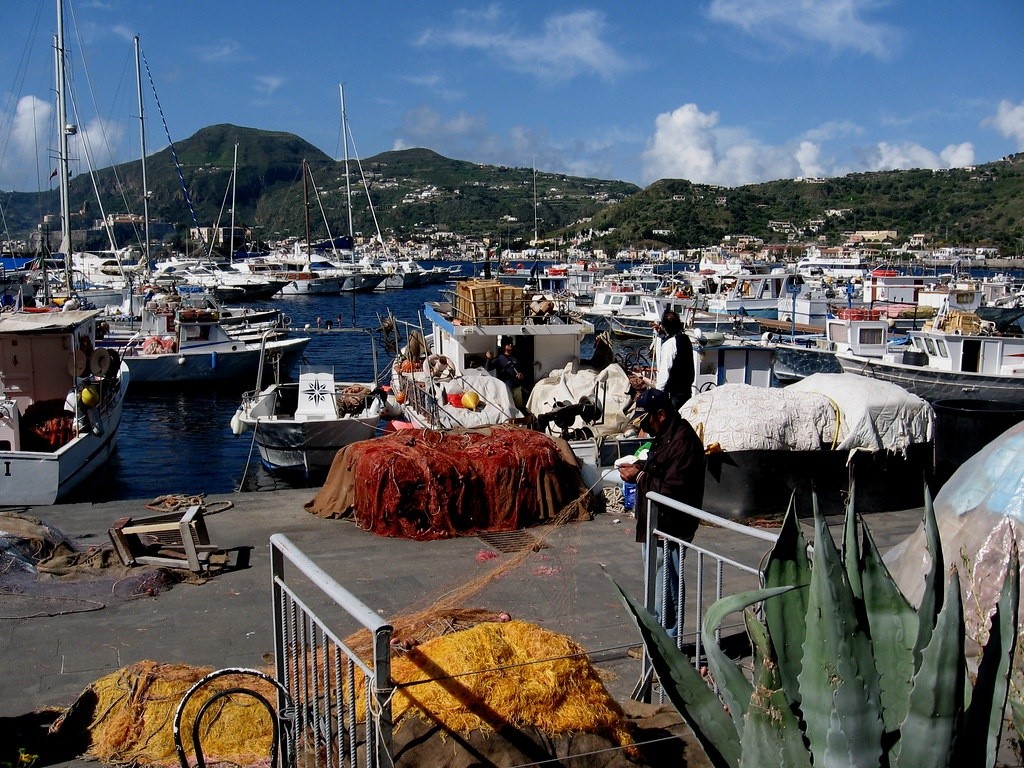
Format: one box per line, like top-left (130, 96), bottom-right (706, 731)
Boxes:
top-left (533, 259), bottom-right (1024, 402)
top-left (93, 288), bottom-right (310, 382)
top-left (0, 310), bottom-right (130, 505)
top-left (239, 327), bottom-right (384, 470)
top-left (387, 300), bottom-right (653, 461)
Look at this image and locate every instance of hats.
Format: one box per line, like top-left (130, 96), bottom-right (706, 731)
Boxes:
top-left (628, 388), bottom-right (671, 425)
top-left (68, 351), bottom-right (86, 376)
top-left (90, 348), bottom-right (111, 375)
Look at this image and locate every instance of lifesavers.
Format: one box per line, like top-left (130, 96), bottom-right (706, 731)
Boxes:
top-left (591, 261), bottom-right (598, 272)
top-left (422, 354), bottom-right (456, 381)
top-left (953, 329), bottom-right (963, 337)
top-left (282, 316), bottom-right (292, 326)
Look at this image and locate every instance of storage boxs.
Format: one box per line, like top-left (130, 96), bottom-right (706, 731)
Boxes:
top-left (883, 352), bottom-right (903, 364)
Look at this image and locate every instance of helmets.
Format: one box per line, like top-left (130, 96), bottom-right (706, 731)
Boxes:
top-left (81, 387), bottom-right (99, 406)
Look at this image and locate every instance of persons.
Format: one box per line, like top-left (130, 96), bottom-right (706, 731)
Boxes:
top-left (617, 386), bottom-right (705, 661)
top-left (484, 336), bottom-right (524, 410)
top-left (654, 309), bottom-right (695, 411)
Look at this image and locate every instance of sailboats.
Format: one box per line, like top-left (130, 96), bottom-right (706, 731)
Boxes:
top-left (0, 0), bottom-right (449, 299)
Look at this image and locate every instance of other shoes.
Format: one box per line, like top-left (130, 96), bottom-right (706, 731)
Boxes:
top-left (628, 646), bottom-right (643, 659)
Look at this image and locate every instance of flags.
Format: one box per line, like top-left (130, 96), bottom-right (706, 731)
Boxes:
top-left (31, 258), bottom-right (41, 272)
